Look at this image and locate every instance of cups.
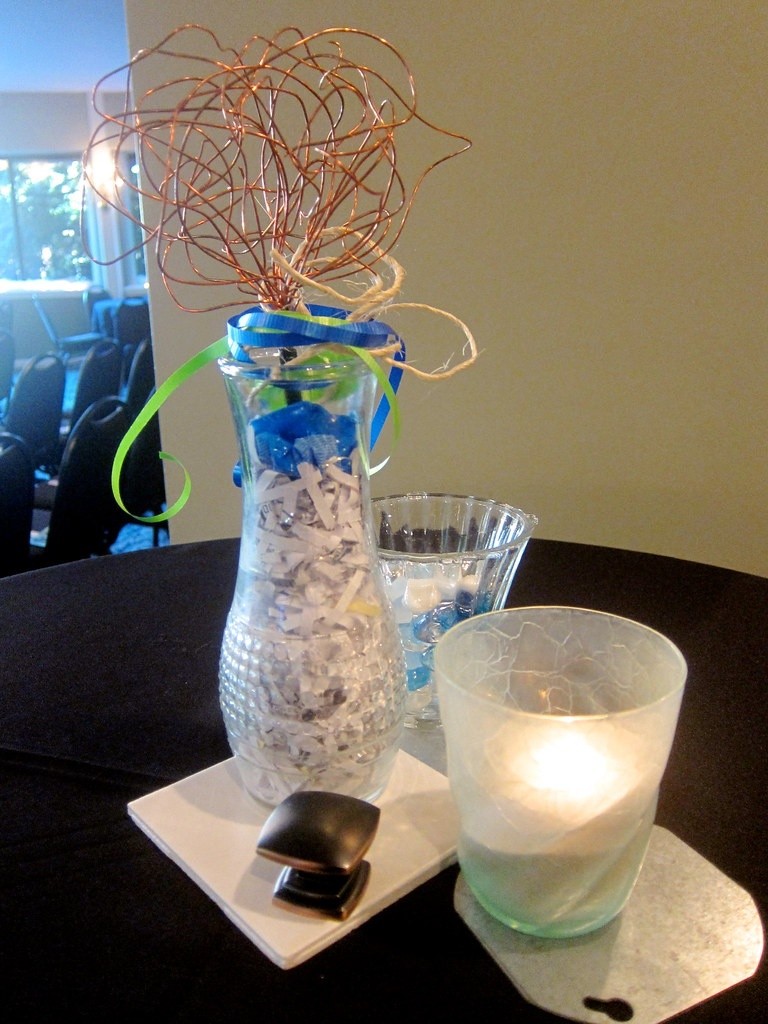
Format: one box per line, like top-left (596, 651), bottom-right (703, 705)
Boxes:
top-left (433, 604), bottom-right (690, 940)
top-left (364, 494), bottom-right (540, 733)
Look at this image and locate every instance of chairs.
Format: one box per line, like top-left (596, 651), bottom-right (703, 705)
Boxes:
top-left (0, 286), bottom-right (169, 576)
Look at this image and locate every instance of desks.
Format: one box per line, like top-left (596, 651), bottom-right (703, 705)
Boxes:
top-left (0, 526), bottom-right (768, 1024)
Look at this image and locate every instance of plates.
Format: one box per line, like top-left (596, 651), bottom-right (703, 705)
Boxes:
top-left (126, 741), bottom-right (461, 973)
top-left (454, 821), bottom-right (764, 1024)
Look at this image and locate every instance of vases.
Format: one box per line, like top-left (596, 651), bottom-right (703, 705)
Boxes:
top-left (217, 345), bottom-right (408, 805)
